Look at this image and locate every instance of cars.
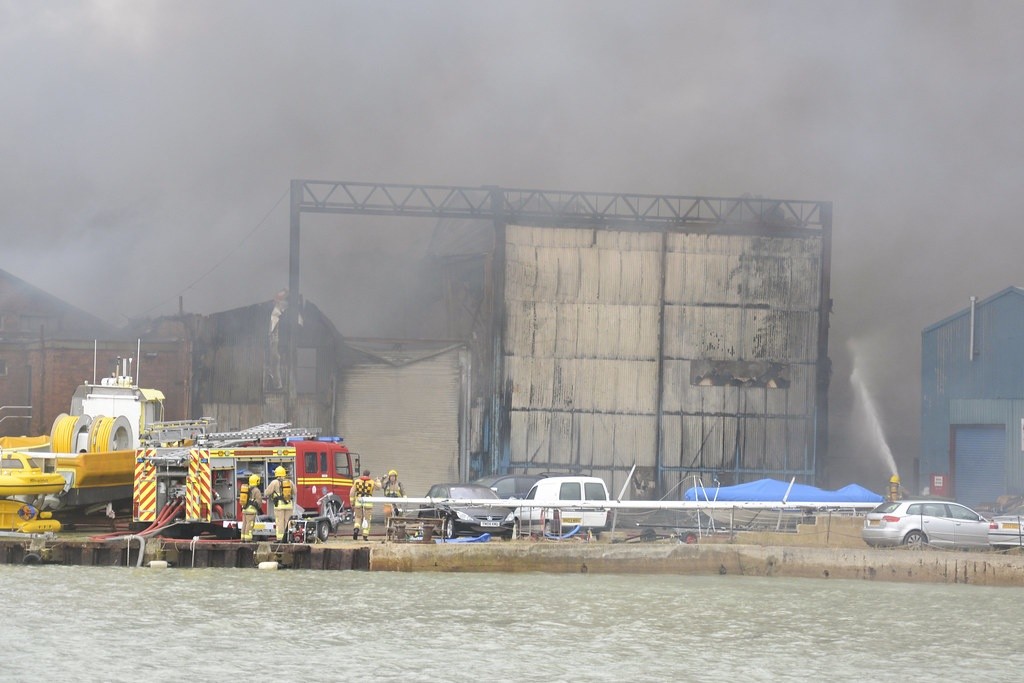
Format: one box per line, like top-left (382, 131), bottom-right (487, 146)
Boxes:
top-left (986, 514), bottom-right (1024, 549)
top-left (860, 499), bottom-right (991, 552)
top-left (420, 482), bottom-right (517, 540)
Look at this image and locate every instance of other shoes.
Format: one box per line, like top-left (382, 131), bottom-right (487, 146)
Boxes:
top-left (353, 529), bottom-right (358, 540)
top-left (364, 536), bottom-right (368, 540)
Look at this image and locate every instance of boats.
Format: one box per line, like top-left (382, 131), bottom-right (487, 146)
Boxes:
top-left (0, 338), bottom-right (219, 514)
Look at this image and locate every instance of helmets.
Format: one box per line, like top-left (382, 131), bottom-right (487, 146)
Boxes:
top-left (249, 474), bottom-right (260, 487)
top-left (388, 470), bottom-right (398, 480)
top-left (275, 466), bottom-right (286, 478)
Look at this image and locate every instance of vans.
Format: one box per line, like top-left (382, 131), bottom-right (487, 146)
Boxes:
top-left (471, 473), bottom-right (551, 509)
top-left (515, 475), bottom-right (612, 536)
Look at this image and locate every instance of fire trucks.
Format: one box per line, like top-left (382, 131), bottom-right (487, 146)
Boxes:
top-left (126, 421), bottom-right (362, 542)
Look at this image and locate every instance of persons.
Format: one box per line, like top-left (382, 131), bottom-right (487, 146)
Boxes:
top-left (885, 475), bottom-right (911, 501)
top-left (380, 469), bottom-right (407, 528)
top-left (264, 466), bottom-right (297, 542)
top-left (240, 475), bottom-right (261, 543)
top-left (349, 470), bottom-right (381, 541)
